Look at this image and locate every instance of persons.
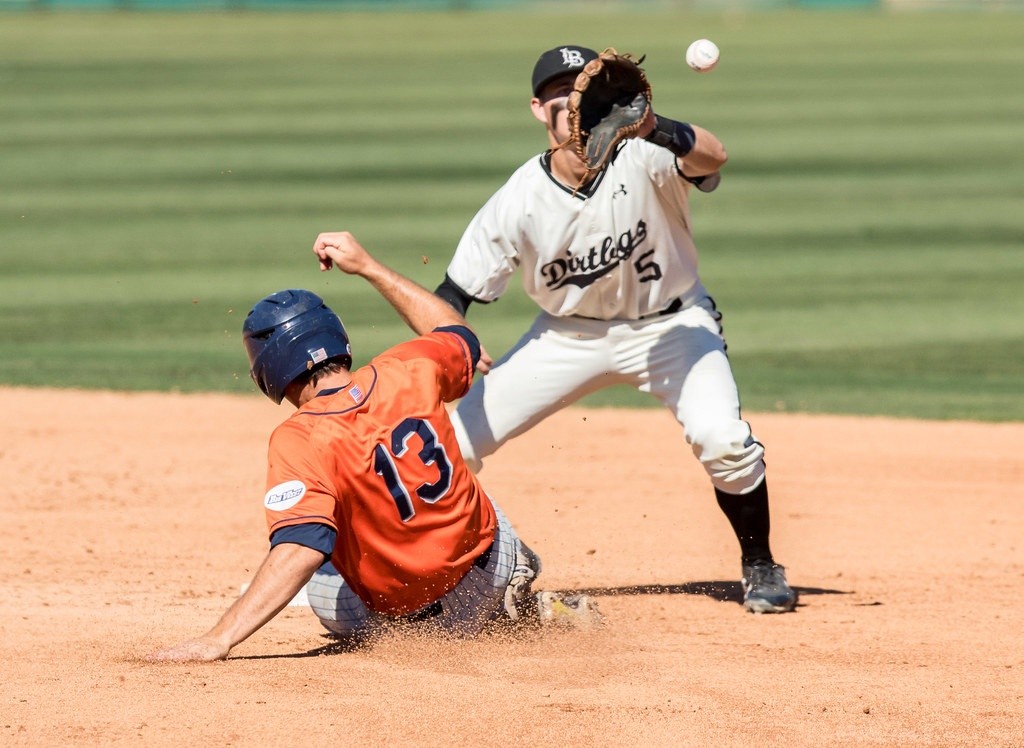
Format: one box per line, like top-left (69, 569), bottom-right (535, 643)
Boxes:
top-left (158, 231), bottom-right (537, 663)
top-left (435, 45), bottom-right (795, 614)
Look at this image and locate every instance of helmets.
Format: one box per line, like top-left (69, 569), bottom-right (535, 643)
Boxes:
top-left (244, 289), bottom-right (354, 405)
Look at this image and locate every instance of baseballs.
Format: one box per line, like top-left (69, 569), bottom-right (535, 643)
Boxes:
top-left (685, 38), bottom-right (720, 73)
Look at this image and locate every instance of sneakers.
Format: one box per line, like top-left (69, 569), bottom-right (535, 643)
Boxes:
top-left (743, 561), bottom-right (796, 613)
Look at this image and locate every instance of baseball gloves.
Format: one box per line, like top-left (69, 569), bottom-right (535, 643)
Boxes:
top-left (566, 52), bottom-right (652, 174)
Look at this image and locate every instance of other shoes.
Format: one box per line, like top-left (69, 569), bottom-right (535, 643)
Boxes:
top-left (530, 590), bottom-right (603, 629)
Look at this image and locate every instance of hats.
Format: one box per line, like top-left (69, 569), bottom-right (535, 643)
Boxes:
top-left (533, 46), bottom-right (599, 97)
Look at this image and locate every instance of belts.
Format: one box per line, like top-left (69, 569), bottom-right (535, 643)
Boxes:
top-left (405, 544), bottom-right (494, 625)
top-left (663, 299), bottom-right (683, 317)
